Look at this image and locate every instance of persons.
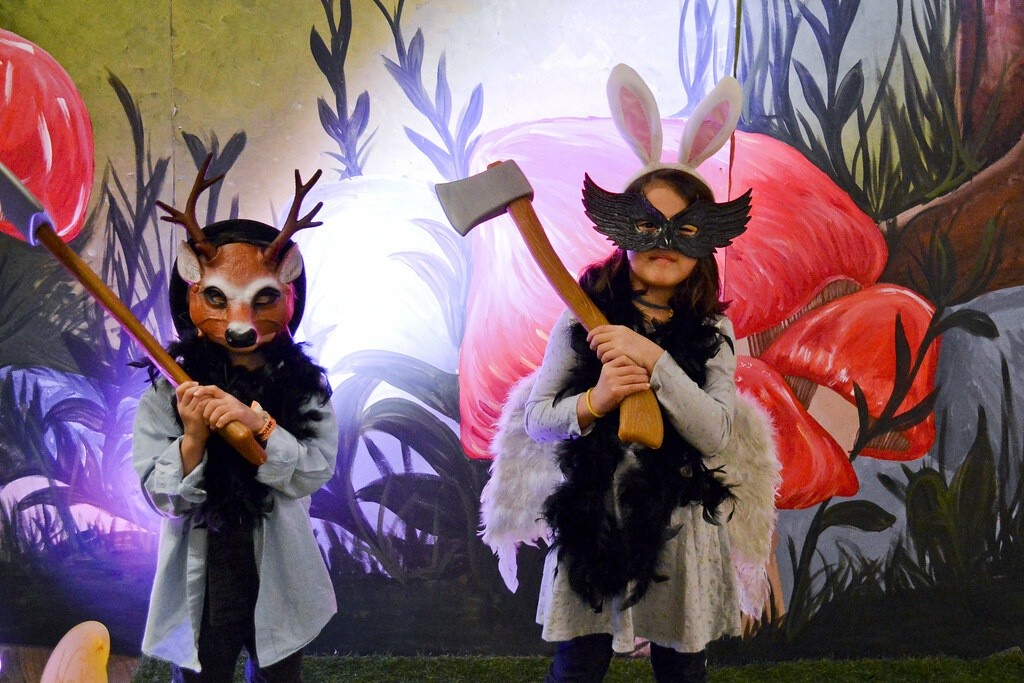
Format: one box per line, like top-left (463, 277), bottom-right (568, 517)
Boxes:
top-left (125, 220), bottom-right (340, 683)
top-left (521, 160), bottom-right (749, 683)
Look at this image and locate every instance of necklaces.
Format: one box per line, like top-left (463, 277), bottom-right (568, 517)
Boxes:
top-left (631, 296), bottom-right (675, 318)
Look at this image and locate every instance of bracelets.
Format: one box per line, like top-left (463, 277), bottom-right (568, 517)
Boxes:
top-left (250, 401), bottom-right (276, 443)
top-left (586, 387), bottom-right (605, 419)
